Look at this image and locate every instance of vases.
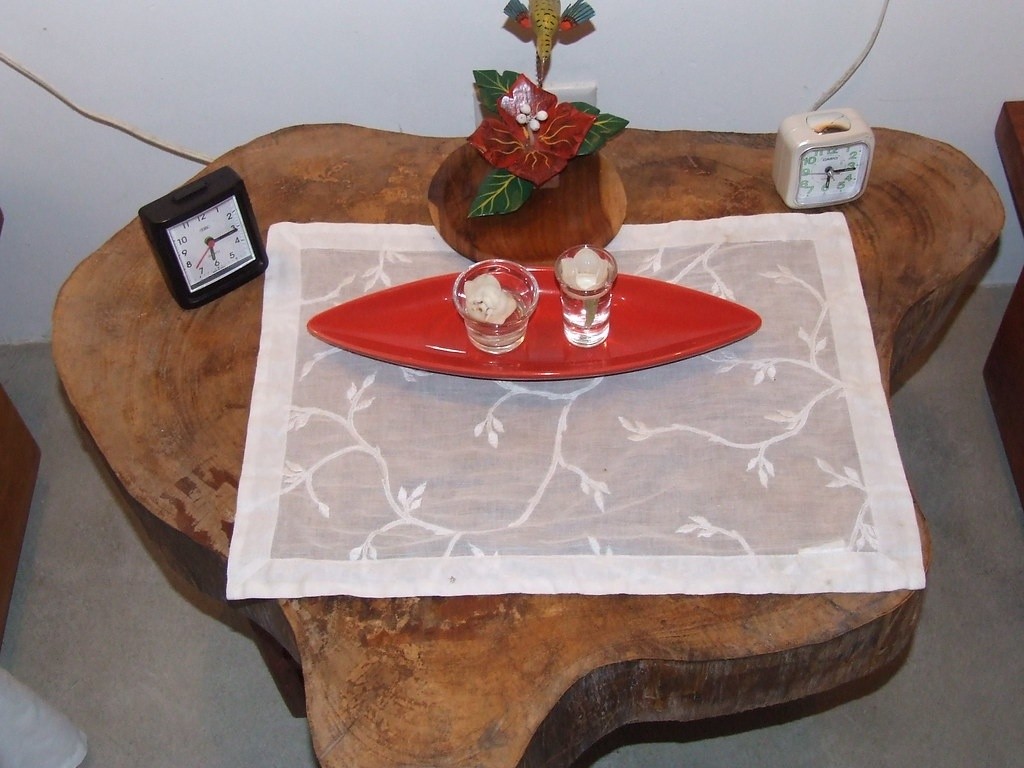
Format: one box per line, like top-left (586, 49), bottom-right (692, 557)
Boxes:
top-left (426, 136), bottom-right (628, 267)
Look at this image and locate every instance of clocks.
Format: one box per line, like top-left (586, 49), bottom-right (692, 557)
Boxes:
top-left (137, 164), bottom-right (270, 312)
top-left (770, 106), bottom-right (876, 210)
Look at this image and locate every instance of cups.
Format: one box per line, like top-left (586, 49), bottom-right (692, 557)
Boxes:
top-left (553, 244), bottom-right (617, 349)
top-left (452, 259), bottom-right (540, 355)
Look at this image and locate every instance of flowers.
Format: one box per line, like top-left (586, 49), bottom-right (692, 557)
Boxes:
top-left (467, 0), bottom-right (630, 220)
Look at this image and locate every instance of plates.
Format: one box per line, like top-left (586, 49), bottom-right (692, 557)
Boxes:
top-left (307, 266), bottom-right (761, 381)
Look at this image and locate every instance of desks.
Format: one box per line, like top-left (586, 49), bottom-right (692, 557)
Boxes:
top-left (47, 113), bottom-right (1005, 768)
